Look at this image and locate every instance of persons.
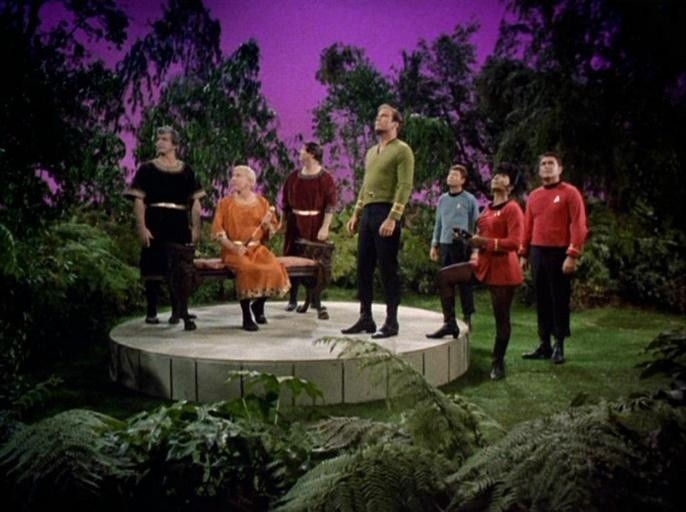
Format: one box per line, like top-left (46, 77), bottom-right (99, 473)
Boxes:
top-left (429, 164), bottom-right (479, 326)
top-left (342, 103), bottom-right (416, 338)
top-left (426, 162), bottom-right (525, 380)
top-left (278, 142), bottom-right (337, 310)
top-left (121, 125), bottom-right (207, 323)
top-left (210, 165), bottom-right (292, 331)
top-left (517, 152), bottom-right (589, 364)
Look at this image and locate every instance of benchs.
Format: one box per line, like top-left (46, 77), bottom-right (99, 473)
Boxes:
top-left (169, 238), bottom-right (335, 331)
